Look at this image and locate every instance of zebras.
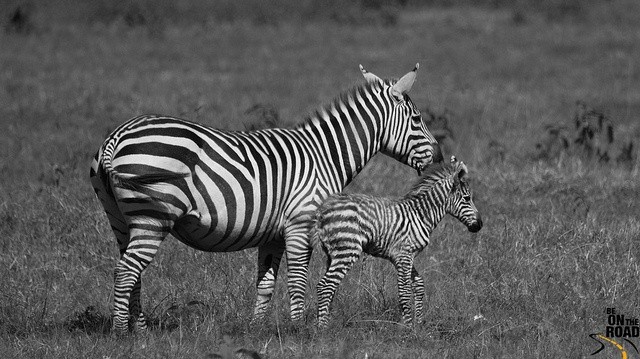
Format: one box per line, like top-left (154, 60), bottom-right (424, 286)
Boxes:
top-left (90, 62), bottom-right (444, 336)
top-left (310, 155), bottom-right (484, 331)
